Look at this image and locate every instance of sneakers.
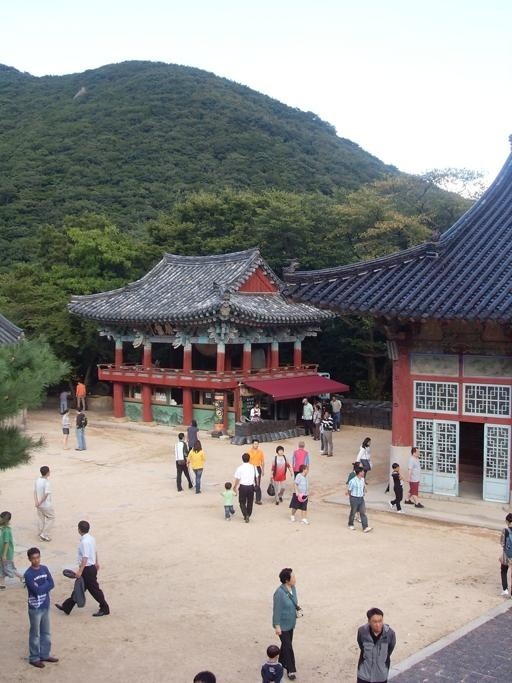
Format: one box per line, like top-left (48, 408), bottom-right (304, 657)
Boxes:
top-left (287, 671), bottom-right (297, 680)
top-left (28, 659), bottom-right (46, 669)
top-left (300, 517), bottom-right (310, 526)
top-left (275, 495), bottom-right (284, 506)
top-left (243, 514), bottom-right (250, 524)
top-left (188, 484), bottom-right (203, 495)
top-left (388, 497), bottom-right (425, 514)
top-left (177, 488), bottom-right (185, 492)
top-left (499, 589), bottom-right (510, 596)
top-left (363, 525), bottom-right (374, 533)
top-left (38, 534), bottom-right (53, 543)
top-left (347, 523), bottom-right (357, 533)
top-left (290, 513), bottom-right (296, 522)
top-left (39, 655), bottom-right (60, 663)
top-left (0, 583), bottom-right (9, 593)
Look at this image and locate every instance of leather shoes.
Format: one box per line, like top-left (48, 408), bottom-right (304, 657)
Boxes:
top-left (54, 602), bottom-right (71, 616)
top-left (92, 610), bottom-right (111, 617)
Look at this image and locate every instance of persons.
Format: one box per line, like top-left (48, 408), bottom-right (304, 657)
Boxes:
top-left (54, 521), bottom-right (109, 617)
top-left (175, 395), bottom-right (424, 533)
top-left (1, 511), bottom-right (25, 590)
top-left (357, 608), bottom-right (394, 683)
top-left (35, 467), bottom-right (54, 542)
top-left (261, 645), bottom-right (283, 683)
top-left (499, 512), bottom-right (512, 595)
top-left (58, 380), bottom-right (88, 451)
top-left (274, 568), bottom-right (303, 680)
top-left (24, 548), bottom-right (59, 668)
top-left (194, 671), bottom-right (215, 682)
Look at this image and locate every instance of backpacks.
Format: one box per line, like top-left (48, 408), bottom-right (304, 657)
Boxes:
top-left (503, 527), bottom-right (512, 559)
top-left (80, 416), bottom-right (88, 428)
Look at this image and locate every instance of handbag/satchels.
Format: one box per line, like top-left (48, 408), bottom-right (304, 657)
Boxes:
top-left (253, 485), bottom-right (260, 493)
top-left (360, 459), bottom-right (371, 470)
top-left (177, 460), bottom-right (186, 466)
top-left (295, 606), bottom-right (304, 619)
top-left (289, 492), bottom-right (309, 511)
top-left (266, 483), bottom-right (277, 497)
